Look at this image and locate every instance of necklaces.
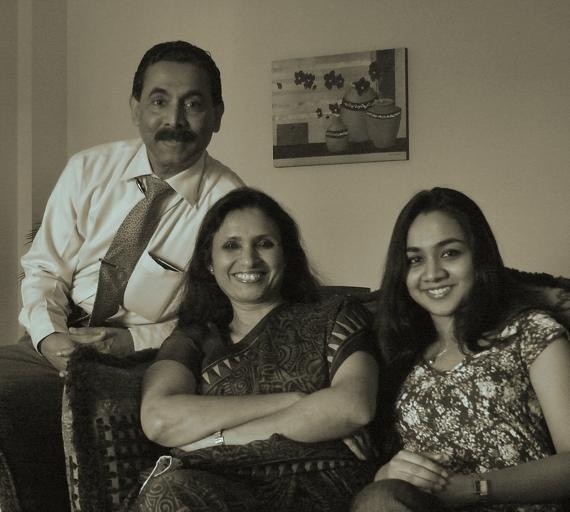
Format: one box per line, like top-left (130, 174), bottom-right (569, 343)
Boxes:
top-left (426, 339), bottom-right (458, 367)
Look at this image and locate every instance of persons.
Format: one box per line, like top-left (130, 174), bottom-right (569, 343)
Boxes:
top-left (350, 188), bottom-right (569, 511)
top-left (135, 188), bottom-right (381, 510)
top-left (0, 40), bottom-right (248, 463)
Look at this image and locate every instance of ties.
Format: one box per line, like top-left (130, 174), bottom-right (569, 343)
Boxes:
top-left (86, 175), bottom-right (174, 328)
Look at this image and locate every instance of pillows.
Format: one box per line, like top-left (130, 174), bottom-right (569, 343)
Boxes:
top-left (61, 346), bottom-right (157, 512)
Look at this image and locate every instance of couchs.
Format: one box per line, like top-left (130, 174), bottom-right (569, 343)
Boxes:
top-left (325, 272), bottom-right (570, 327)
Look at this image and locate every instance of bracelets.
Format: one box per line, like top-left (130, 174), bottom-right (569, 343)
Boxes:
top-left (212, 428), bottom-right (226, 447)
top-left (474, 469), bottom-right (492, 505)
